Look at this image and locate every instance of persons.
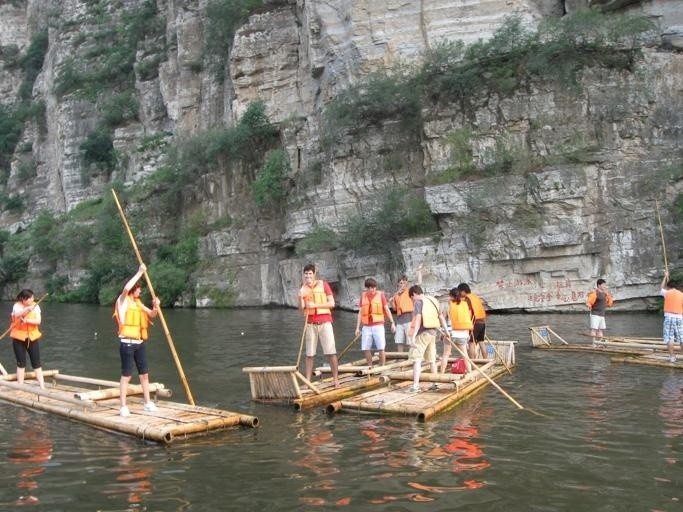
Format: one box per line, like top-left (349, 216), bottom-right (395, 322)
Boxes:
top-left (353, 278), bottom-right (396, 366)
top-left (388, 261), bottom-right (423, 352)
top-left (457, 282), bottom-right (488, 360)
top-left (584, 278), bottom-right (613, 350)
top-left (296, 263), bottom-right (341, 389)
top-left (439, 287), bottom-right (472, 374)
top-left (405, 284), bottom-right (450, 394)
top-left (7, 288), bottom-right (45, 391)
top-left (658, 268), bottom-right (683, 364)
top-left (111, 262), bottom-right (161, 417)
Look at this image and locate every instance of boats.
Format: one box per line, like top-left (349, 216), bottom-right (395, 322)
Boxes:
top-left (0, 369), bottom-right (259, 447)
top-left (240, 337), bottom-right (517, 425)
top-left (527, 324), bottom-right (683, 371)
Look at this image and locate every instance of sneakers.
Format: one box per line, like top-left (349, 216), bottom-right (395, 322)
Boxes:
top-left (403, 386), bottom-right (421, 394)
top-left (428, 383), bottom-right (440, 391)
top-left (119, 405), bottom-right (132, 417)
top-left (143, 401), bottom-right (161, 413)
top-left (668, 356), bottom-right (676, 363)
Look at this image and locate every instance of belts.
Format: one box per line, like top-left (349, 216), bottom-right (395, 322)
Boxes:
top-left (307, 321), bottom-right (327, 326)
top-left (120, 338), bottom-right (143, 345)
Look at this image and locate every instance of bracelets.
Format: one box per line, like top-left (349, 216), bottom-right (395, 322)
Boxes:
top-left (25, 319), bottom-right (27, 323)
top-left (389, 319), bottom-right (394, 323)
